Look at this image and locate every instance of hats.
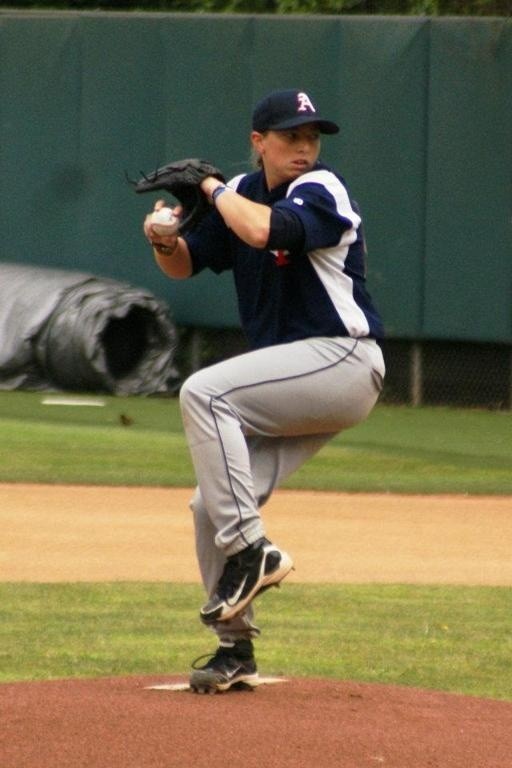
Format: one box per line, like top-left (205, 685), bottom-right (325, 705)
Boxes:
top-left (250, 85), bottom-right (341, 136)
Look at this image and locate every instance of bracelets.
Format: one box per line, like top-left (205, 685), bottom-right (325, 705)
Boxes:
top-left (150, 239), bottom-right (181, 258)
top-left (210, 185), bottom-right (230, 203)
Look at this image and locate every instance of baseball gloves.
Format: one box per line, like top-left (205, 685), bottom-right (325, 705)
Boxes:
top-left (135, 160), bottom-right (225, 239)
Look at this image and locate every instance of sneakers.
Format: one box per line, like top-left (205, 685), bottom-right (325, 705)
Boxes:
top-left (186, 640), bottom-right (265, 695)
top-left (199, 534), bottom-right (294, 628)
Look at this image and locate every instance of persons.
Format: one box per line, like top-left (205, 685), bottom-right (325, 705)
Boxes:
top-left (131, 87), bottom-right (388, 700)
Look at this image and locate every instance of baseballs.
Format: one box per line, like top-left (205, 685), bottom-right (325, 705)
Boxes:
top-left (150, 205), bottom-right (180, 237)
